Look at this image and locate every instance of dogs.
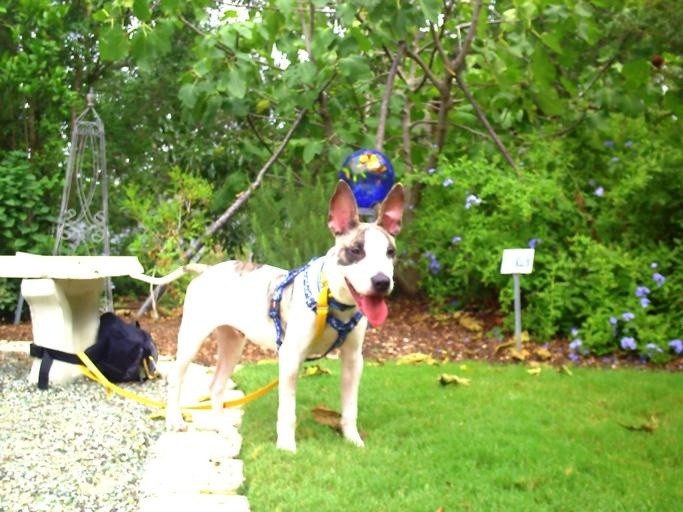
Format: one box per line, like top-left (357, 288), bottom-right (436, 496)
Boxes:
top-left (128, 178), bottom-right (407, 456)
